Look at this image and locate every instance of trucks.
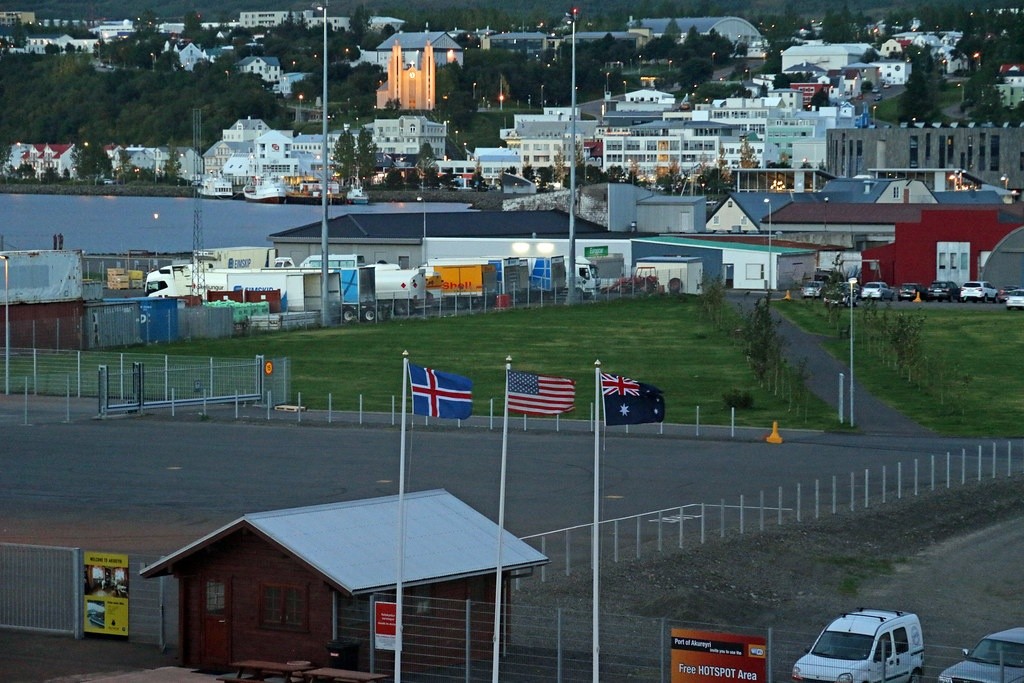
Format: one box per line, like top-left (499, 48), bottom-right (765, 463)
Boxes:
top-left (485, 258), bottom-right (603, 299)
top-left (142, 262), bottom-right (343, 326)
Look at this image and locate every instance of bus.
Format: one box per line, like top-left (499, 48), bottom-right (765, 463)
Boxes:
top-left (860, 281), bottom-right (896, 302)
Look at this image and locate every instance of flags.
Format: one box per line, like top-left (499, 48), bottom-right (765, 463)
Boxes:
top-left (601, 373), bottom-right (664, 426)
top-left (408, 363), bottom-right (472, 420)
top-left (508, 370), bottom-right (576, 417)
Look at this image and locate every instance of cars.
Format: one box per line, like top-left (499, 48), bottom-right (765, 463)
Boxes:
top-left (934, 625), bottom-right (1024, 683)
top-left (1005, 288), bottom-right (1024, 311)
top-left (103, 179), bottom-right (115, 184)
top-left (897, 283), bottom-right (929, 302)
top-left (998, 285), bottom-right (1019, 303)
top-left (800, 280), bottom-right (828, 299)
top-left (822, 284), bottom-right (859, 308)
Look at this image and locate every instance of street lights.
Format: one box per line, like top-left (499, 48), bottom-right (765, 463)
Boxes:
top-left (999, 172), bottom-right (1010, 190)
top-left (310, 0), bottom-right (330, 325)
top-left (763, 198), bottom-right (773, 296)
top-left (565, 18), bottom-right (577, 303)
top-left (849, 275), bottom-right (857, 427)
top-left (0, 255), bottom-right (11, 398)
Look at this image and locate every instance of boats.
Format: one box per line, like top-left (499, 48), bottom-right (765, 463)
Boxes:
top-left (345, 167), bottom-right (370, 203)
top-left (198, 170), bottom-right (234, 199)
top-left (242, 167), bottom-right (289, 204)
top-left (284, 179), bottom-right (346, 205)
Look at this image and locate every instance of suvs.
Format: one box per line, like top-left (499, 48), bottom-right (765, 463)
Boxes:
top-left (791, 606), bottom-right (925, 683)
top-left (959, 281), bottom-right (1000, 303)
top-left (932, 281), bottom-right (960, 300)
